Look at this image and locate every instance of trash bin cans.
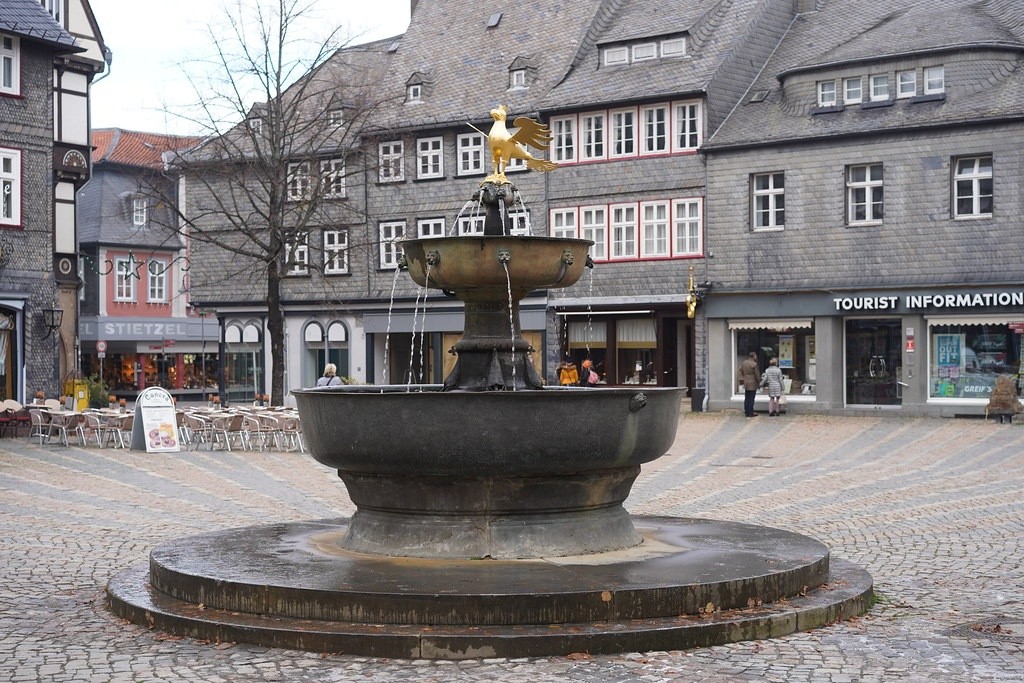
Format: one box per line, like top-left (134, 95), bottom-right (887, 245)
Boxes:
top-left (690, 387), bottom-right (706, 412)
top-left (63, 379), bottom-right (89, 411)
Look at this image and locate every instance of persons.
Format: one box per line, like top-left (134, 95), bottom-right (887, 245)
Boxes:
top-left (760, 356), bottom-right (787, 417)
top-left (577, 360), bottom-right (594, 387)
top-left (740, 352), bottom-right (762, 419)
top-left (316, 364), bottom-right (346, 388)
top-left (560, 358), bottom-right (580, 388)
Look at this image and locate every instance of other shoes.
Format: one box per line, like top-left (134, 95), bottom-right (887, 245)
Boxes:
top-left (748, 414), bottom-right (758, 417)
top-left (776, 412), bottom-right (779, 416)
top-left (769, 412), bottom-right (775, 416)
top-left (745, 414), bottom-right (748, 417)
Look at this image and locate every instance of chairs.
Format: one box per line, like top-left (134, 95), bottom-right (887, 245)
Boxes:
top-left (0, 399), bottom-right (304, 454)
